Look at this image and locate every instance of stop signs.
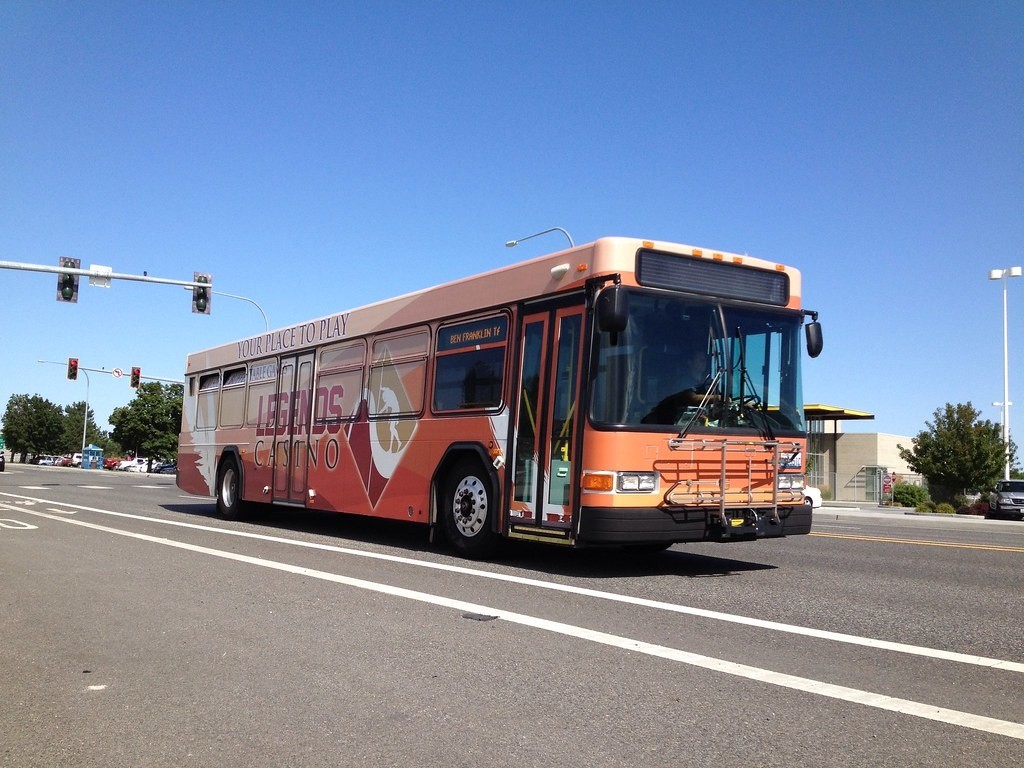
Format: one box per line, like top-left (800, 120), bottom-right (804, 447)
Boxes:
top-left (884, 477), bottom-right (891, 485)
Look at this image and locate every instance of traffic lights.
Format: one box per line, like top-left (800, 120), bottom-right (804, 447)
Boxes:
top-left (67, 357), bottom-right (79, 382)
top-left (130, 366), bottom-right (141, 387)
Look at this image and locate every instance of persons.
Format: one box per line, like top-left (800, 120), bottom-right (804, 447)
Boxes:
top-left (684, 351), bottom-right (733, 416)
top-left (125, 454), bottom-right (131, 461)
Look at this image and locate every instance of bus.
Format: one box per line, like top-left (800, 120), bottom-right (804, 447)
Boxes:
top-left (176, 237), bottom-right (826, 558)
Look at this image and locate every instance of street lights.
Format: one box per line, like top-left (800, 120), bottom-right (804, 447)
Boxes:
top-left (988, 266), bottom-right (1023, 479)
top-left (36, 359), bottom-right (89, 467)
top-left (506, 227), bottom-right (574, 247)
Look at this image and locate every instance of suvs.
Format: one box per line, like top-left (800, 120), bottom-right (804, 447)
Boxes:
top-left (988, 479), bottom-right (1024, 518)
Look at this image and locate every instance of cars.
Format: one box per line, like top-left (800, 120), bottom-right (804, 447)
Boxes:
top-left (801, 484), bottom-right (822, 509)
top-left (30, 452), bottom-right (177, 474)
top-left (0, 451), bottom-right (6, 472)
